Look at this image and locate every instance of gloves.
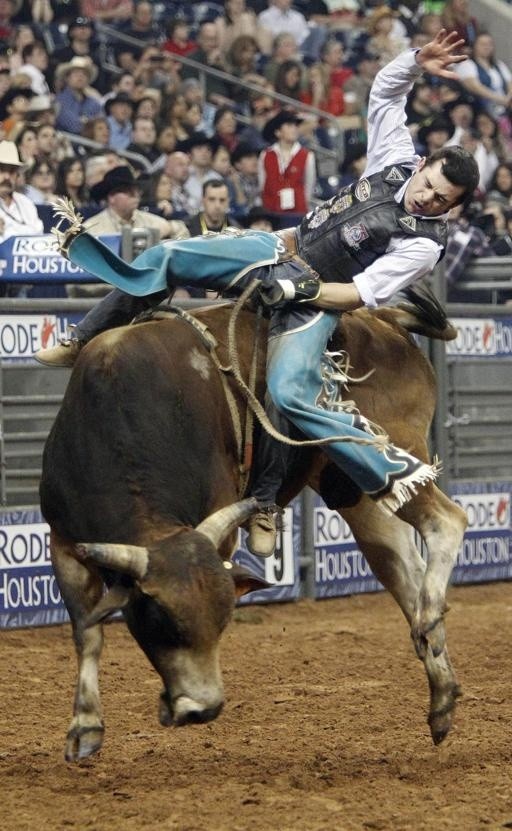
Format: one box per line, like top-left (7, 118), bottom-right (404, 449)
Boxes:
top-left (249, 277), bottom-right (321, 310)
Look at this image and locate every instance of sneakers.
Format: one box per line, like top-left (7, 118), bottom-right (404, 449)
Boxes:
top-left (245, 509), bottom-right (280, 558)
top-left (30, 337), bottom-right (75, 369)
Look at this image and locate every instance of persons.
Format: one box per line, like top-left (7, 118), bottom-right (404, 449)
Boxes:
top-left (0, 0), bottom-right (509, 302)
top-left (31, 22), bottom-right (482, 561)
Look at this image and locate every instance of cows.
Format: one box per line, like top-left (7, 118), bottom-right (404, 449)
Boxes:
top-left (33, 278), bottom-right (477, 764)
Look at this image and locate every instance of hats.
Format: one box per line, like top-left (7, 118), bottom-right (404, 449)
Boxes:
top-left (87, 164), bottom-right (146, 200)
top-left (104, 90), bottom-right (138, 116)
top-left (66, 15), bottom-right (96, 42)
top-left (231, 142), bottom-right (265, 163)
top-left (54, 54), bottom-right (97, 80)
top-left (262, 109), bottom-right (305, 143)
top-left (0, 140), bottom-right (26, 167)
top-left (184, 134), bottom-right (221, 154)
